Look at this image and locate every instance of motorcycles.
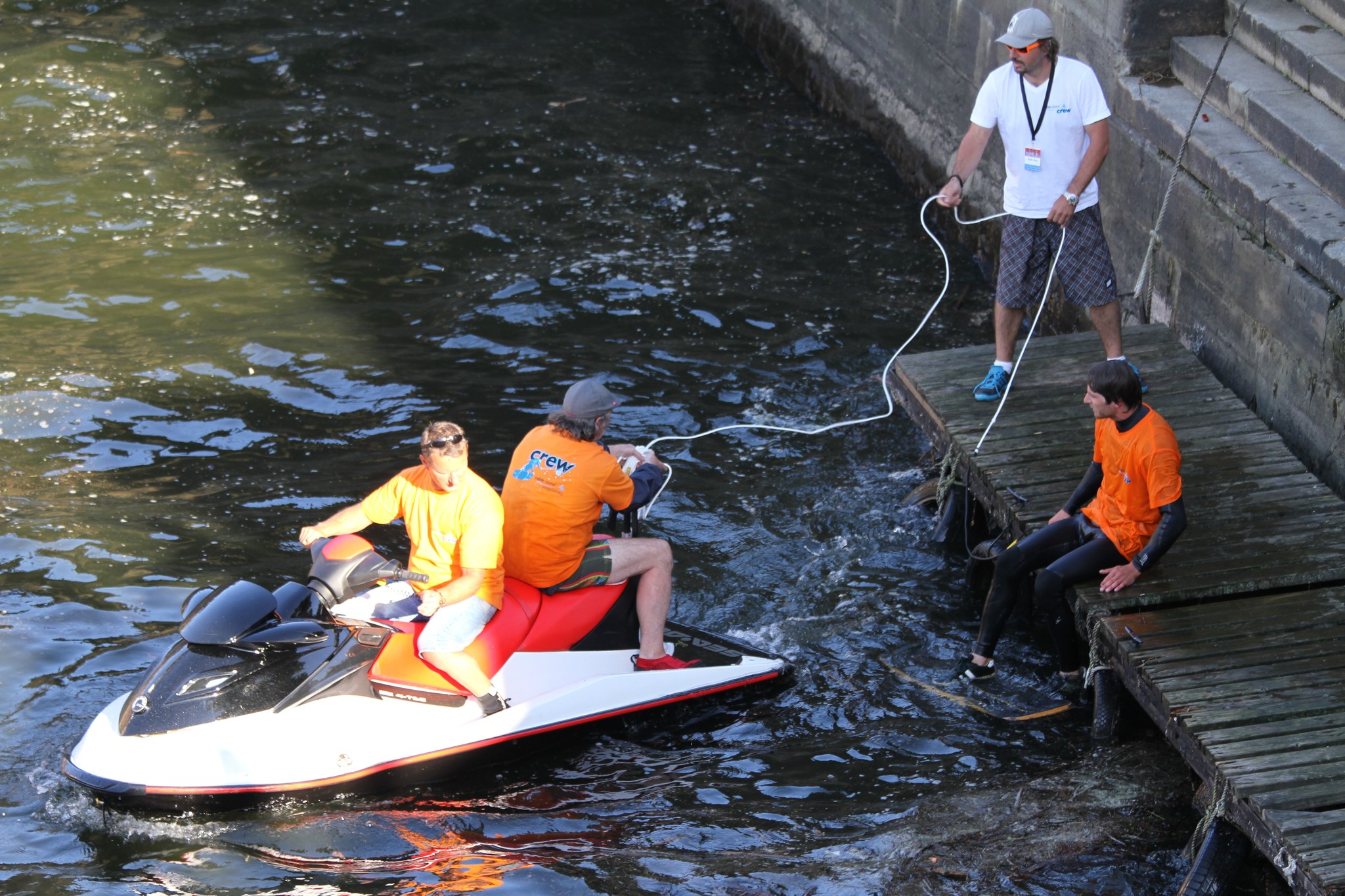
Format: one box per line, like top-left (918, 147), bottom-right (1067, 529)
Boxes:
top-left (59, 534), bottom-right (799, 815)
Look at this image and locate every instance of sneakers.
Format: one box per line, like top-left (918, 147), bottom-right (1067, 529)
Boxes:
top-left (477, 692), bottom-right (512, 717)
top-left (972, 365), bottom-right (1015, 401)
top-left (1050, 668), bottom-right (1086, 688)
top-left (1122, 359), bottom-right (1148, 393)
top-left (631, 654), bottom-right (704, 670)
top-left (932, 653), bottom-right (995, 685)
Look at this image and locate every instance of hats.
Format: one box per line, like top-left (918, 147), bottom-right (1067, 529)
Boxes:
top-left (994, 8), bottom-right (1052, 47)
top-left (562, 379), bottom-right (621, 418)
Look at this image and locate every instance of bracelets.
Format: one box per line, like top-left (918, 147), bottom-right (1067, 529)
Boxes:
top-left (949, 173), bottom-right (965, 192)
top-left (430, 587), bottom-right (446, 608)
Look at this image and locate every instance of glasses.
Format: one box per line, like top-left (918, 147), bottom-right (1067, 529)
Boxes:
top-left (420, 433), bottom-right (467, 448)
top-left (1006, 41), bottom-right (1045, 55)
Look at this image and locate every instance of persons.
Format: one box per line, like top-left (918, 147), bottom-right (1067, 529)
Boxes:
top-left (298, 421), bottom-right (512, 717)
top-left (937, 7), bottom-right (1151, 402)
top-left (500, 378), bottom-right (703, 673)
top-left (931, 360), bottom-right (1187, 706)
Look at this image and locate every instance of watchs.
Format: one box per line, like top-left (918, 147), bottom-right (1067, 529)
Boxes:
top-left (1062, 191), bottom-right (1079, 206)
